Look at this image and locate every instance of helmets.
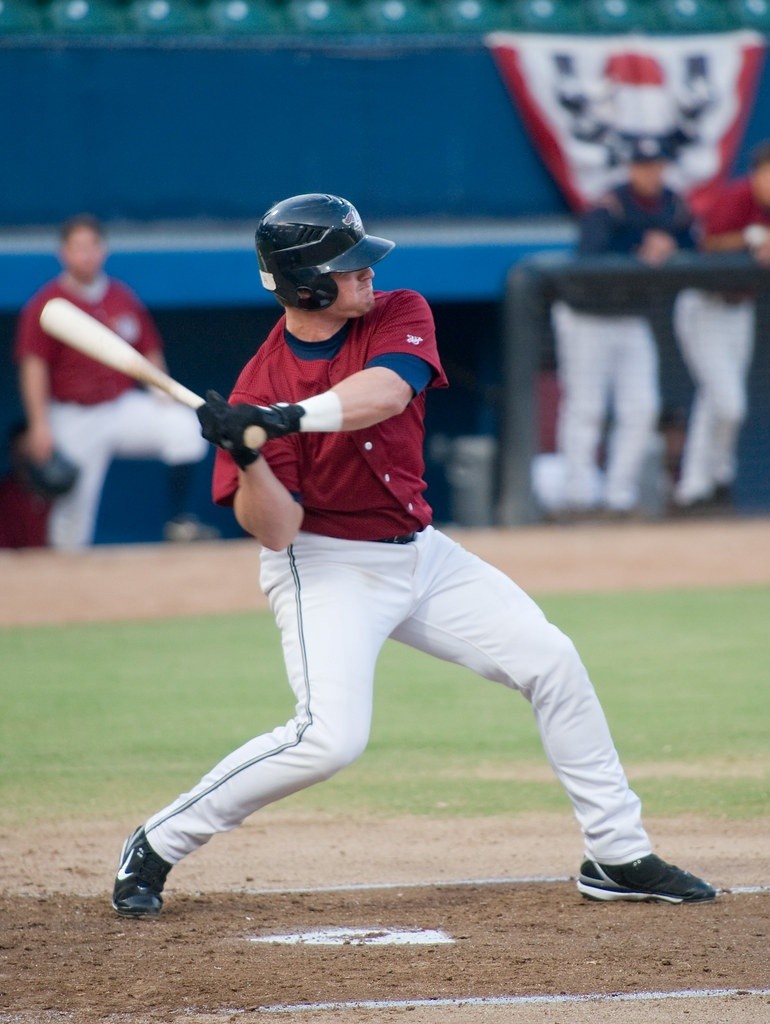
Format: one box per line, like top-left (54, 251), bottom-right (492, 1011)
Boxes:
top-left (255, 193), bottom-right (396, 312)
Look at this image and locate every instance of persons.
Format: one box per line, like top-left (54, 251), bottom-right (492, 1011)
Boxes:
top-left (671, 144), bottom-right (770, 511)
top-left (113, 192), bottom-right (717, 919)
top-left (552, 139), bottom-right (696, 518)
top-left (16, 213), bottom-right (218, 545)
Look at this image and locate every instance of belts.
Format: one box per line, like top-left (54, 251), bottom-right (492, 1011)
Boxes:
top-left (373, 531), bottom-right (416, 544)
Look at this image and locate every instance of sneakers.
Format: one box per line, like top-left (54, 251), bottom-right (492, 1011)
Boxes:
top-left (112, 825), bottom-right (173, 918)
top-left (577, 853), bottom-right (715, 904)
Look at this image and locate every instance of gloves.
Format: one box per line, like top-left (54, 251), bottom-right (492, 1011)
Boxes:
top-left (196, 390), bottom-right (306, 472)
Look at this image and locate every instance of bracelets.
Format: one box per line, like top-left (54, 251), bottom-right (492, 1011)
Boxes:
top-left (298, 391), bottom-right (342, 432)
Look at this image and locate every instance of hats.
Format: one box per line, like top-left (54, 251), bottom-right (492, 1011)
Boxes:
top-left (633, 138), bottom-right (672, 163)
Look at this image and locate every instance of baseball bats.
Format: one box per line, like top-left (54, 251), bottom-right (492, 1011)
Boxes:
top-left (37, 298), bottom-right (265, 451)
